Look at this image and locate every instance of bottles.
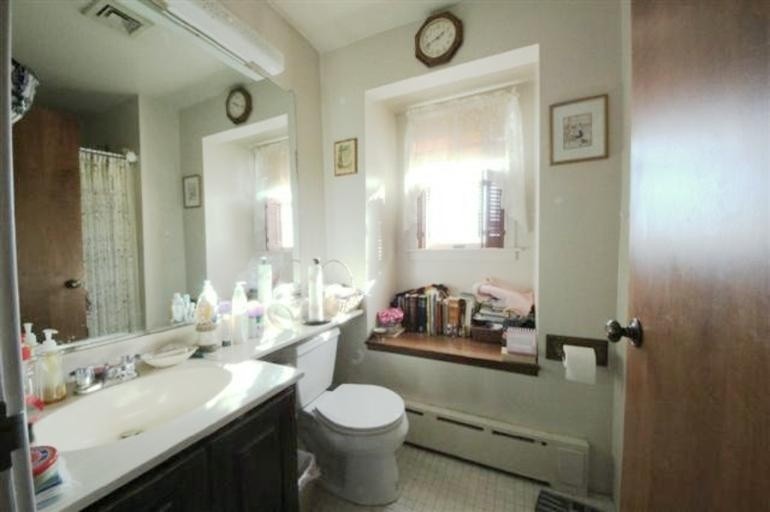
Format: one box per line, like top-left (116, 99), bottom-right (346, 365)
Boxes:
top-left (20, 333), bottom-right (32, 361)
top-left (21, 347), bottom-right (44, 422)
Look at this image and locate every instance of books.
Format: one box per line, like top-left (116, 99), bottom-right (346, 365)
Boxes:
top-left (394, 282), bottom-right (537, 364)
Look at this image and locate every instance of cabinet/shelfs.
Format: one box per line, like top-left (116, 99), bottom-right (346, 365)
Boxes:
top-left (79, 383), bottom-right (299, 512)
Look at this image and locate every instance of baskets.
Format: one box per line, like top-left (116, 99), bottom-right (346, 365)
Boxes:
top-left (471, 322), bottom-right (503, 343)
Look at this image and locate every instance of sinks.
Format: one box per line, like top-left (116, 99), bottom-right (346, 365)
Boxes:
top-left (28, 358), bottom-right (233, 452)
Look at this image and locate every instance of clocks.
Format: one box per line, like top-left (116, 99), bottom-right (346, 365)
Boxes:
top-left (225, 85), bottom-right (252, 124)
top-left (413, 11), bottom-right (464, 69)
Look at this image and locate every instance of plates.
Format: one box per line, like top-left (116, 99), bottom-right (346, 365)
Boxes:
top-left (142, 344), bottom-right (198, 368)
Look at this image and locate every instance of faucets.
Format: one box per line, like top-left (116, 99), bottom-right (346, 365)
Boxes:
top-left (102, 356), bottom-right (128, 381)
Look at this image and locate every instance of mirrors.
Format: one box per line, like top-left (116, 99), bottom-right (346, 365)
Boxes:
top-left (11, 0), bottom-right (302, 366)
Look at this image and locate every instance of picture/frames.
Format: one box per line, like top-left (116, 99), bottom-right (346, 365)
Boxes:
top-left (548, 92), bottom-right (611, 166)
top-left (332, 138), bottom-right (358, 176)
top-left (182, 173), bottom-right (201, 208)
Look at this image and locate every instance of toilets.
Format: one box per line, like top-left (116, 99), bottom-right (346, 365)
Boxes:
top-left (276, 327), bottom-right (408, 506)
